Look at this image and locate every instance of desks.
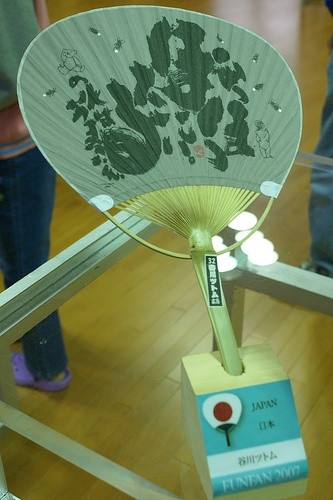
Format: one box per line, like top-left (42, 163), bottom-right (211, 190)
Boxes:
top-left (2, 147), bottom-right (333, 500)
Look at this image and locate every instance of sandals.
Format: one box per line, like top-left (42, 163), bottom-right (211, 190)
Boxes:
top-left (12, 351), bottom-right (71, 392)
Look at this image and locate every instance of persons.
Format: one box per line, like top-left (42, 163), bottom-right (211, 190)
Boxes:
top-left (301, 1), bottom-right (333, 280)
top-left (1, 1), bottom-right (74, 392)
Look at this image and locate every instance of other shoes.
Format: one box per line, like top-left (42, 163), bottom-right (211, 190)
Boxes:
top-left (299, 261), bottom-right (333, 281)
